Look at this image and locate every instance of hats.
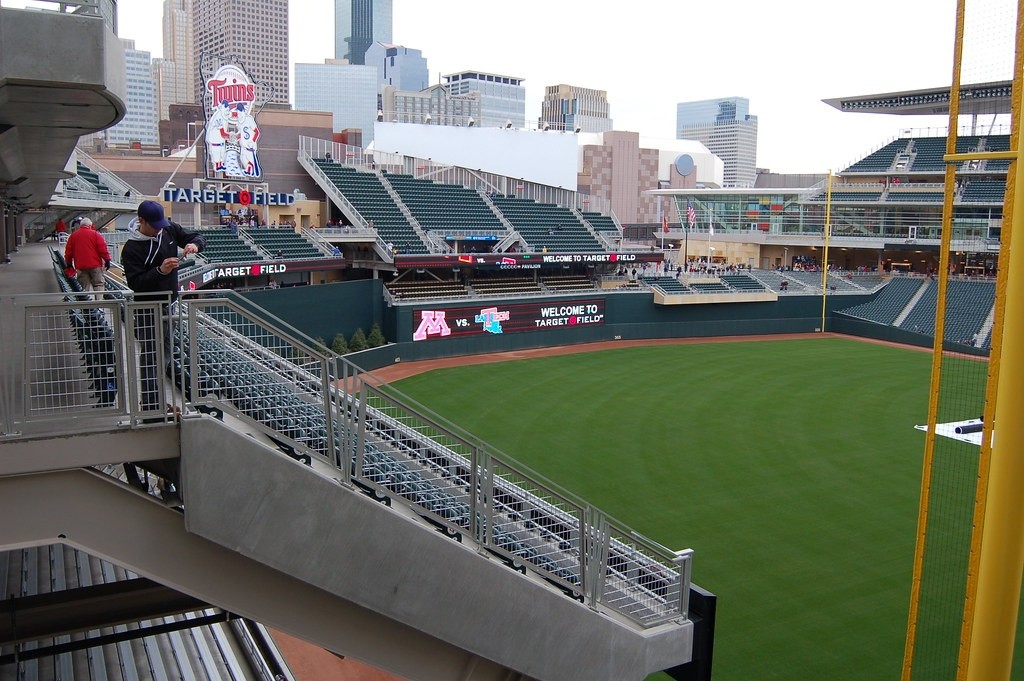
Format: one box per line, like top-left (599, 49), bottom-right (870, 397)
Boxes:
top-left (138, 200), bottom-right (172, 229)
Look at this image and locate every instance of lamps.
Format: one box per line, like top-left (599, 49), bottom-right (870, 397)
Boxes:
top-left (424, 113), bottom-right (432, 124)
top-left (573, 125), bottom-right (582, 134)
top-left (468, 117), bottom-right (475, 128)
top-left (377, 109), bottom-right (384, 122)
top-left (505, 120), bottom-right (512, 130)
top-left (542, 122), bottom-right (549, 132)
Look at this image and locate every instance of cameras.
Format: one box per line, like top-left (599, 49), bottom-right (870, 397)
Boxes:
top-left (178, 254), bottom-right (187, 262)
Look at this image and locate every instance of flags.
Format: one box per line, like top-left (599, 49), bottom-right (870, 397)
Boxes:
top-left (687, 201), bottom-right (696, 226)
top-left (664, 217), bottom-right (670, 234)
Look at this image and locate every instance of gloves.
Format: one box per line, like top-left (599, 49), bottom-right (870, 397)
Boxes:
top-left (67, 265), bottom-right (75, 276)
top-left (105, 261), bottom-right (110, 271)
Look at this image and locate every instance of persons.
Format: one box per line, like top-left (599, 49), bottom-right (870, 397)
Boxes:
top-left (331, 246), bottom-right (339, 258)
top-left (386, 240), bottom-right (412, 259)
top-left (268, 279), bottom-right (277, 290)
top-left (310, 219), bottom-right (343, 230)
top-left (65, 217), bottom-right (111, 315)
top-left (222, 217), bottom-right (296, 229)
top-left (120, 200), bottom-right (206, 408)
top-left (56, 218), bottom-right (67, 240)
top-left (72, 219), bottom-right (77, 233)
top-left (772, 148), bottom-right (1006, 291)
top-left (616, 259), bottom-right (752, 279)
top-left (547, 223), bottom-right (562, 235)
top-left (460, 244), bottom-right (547, 253)
